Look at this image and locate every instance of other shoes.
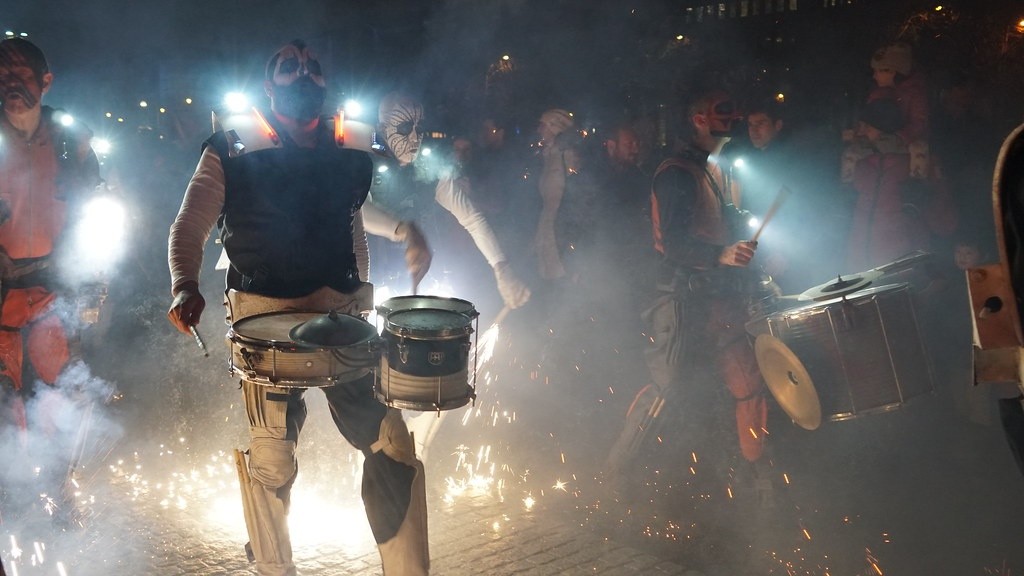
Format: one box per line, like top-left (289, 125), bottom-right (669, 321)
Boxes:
top-left (601, 467), bottom-right (636, 504)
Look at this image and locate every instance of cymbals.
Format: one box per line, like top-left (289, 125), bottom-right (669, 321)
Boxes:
top-left (287, 312), bottom-right (377, 350)
top-left (798, 270), bottom-right (884, 301)
top-left (753, 334), bottom-right (825, 431)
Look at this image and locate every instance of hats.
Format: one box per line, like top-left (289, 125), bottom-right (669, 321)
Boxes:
top-left (870, 42), bottom-right (914, 76)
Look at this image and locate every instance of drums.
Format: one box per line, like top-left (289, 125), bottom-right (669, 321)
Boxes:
top-left (228, 313), bottom-right (374, 388)
top-left (744, 249), bottom-right (957, 425)
top-left (376, 294), bottom-right (478, 416)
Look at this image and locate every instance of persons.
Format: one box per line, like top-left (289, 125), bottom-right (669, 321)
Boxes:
top-left (167, 42), bottom-right (435, 576)
top-left (0, 36), bottom-right (116, 535)
top-left (448, 38), bottom-right (1023, 414)
top-left (596, 92), bottom-right (787, 510)
top-left (361, 88), bottom-right (531, 310)
